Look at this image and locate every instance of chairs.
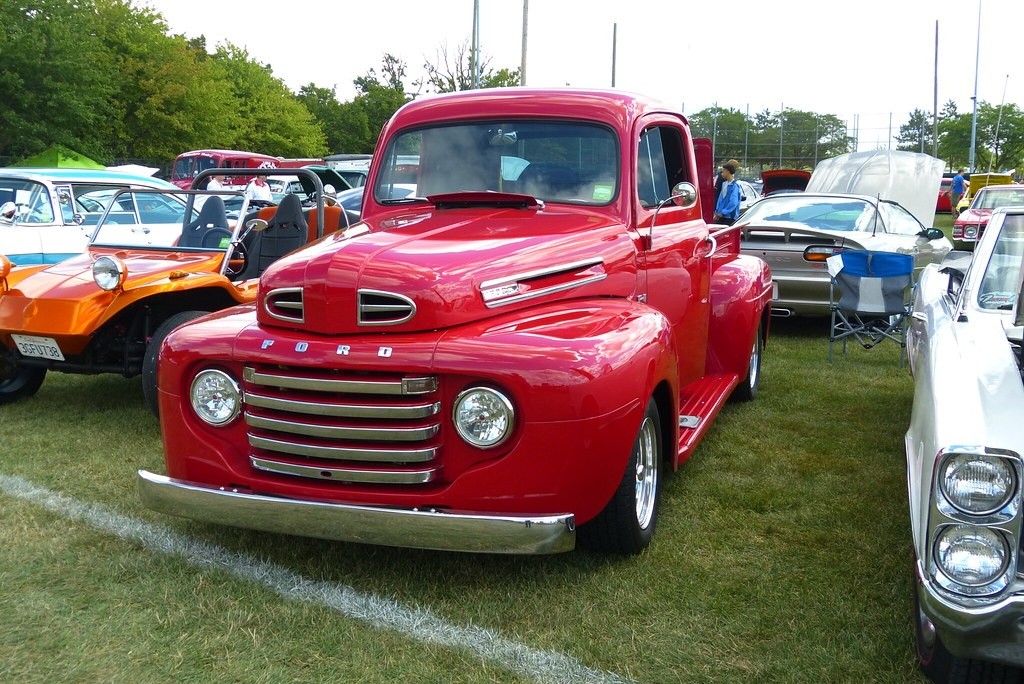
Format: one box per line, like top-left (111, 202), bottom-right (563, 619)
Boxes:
top-left (514, 162), bottom-right (583, 199)
top-left (235, 195), bottom-right (308, 281)
top-left (177, 196), bottom-right (231, 249)
top-left (827, 249), bottom-right (917, 367)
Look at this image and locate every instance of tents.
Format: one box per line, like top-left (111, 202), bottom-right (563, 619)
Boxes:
top-left (0, 143), bottom-right (109, 169)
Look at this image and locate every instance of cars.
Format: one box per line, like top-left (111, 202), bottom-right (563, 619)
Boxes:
top-left (0, 147), bottom-right (532, 406)
top-left (900, 202), bottom-right (1023, 677)
top-left (936, 177), bottom-right (972, 212)
top-left (139, 86), bottom-right (773, 557)
top-left (722, 193), bottom-right (953, 328)
top-left (950, 185), bottom-right (1024, 252)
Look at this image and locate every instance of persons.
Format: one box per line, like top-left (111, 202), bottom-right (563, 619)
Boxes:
top-left (246, 175), bottom-right (273, 203)
top-left (206, 176), bottom-right (227, 191)
top-left (713, 159), bottom-right (740, 214)
top-left (713, 164), bottom-right (741, 226)
top-left (945, 169), bottom-right (968, 219)
